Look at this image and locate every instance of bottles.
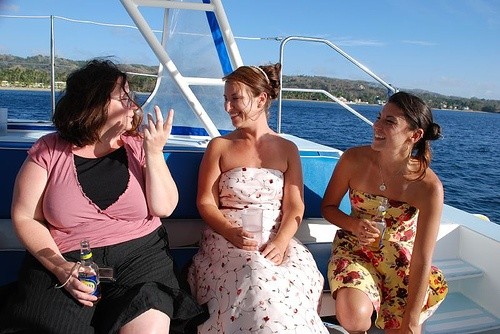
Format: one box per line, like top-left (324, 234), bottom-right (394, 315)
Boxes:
top-left (364, 197), bottom-right (388, 250)
top-left (78, 240), bottom-right (102, 305)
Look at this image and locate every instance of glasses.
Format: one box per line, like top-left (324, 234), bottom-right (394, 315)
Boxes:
top-left (110, 91), bottom-right (132, 106)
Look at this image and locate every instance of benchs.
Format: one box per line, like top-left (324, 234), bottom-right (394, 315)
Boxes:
top-left (0, 240), bottom-right (483, 311)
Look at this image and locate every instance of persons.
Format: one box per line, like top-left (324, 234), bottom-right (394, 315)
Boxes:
top-left (188, 63), bottom-right (331, 334)
top-left (322, 91), bottom-right (448, 334)
top-left (3, 55), bottom-right (203, 334)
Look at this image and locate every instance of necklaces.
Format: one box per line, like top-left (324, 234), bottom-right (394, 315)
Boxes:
top-left (380, 155), bottom-right (410, 192)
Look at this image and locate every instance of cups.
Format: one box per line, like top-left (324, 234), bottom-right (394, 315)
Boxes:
top-left (240, 208), bottom-right (263, 248)
